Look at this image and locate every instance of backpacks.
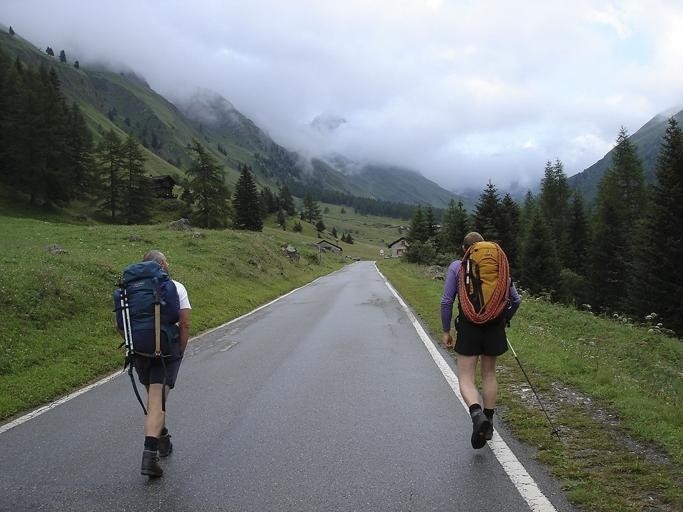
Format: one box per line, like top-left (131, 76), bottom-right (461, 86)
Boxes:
top-left (461, 241), bottom-right (509, 320)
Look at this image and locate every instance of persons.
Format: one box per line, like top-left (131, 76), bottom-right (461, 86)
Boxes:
top-left (438, 230), bottom-right (521, 449)
top-left (115, 250), bottom-right (192, 481)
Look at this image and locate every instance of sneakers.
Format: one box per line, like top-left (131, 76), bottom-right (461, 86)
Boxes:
top-left (141, 450), bottom-right (163, 477)
top-left (471, 413), bottom-right (494, 449)
top-left (159, 433), bottom-right (172, 457)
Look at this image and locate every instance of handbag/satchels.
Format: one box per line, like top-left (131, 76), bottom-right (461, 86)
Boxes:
top-left (113, 261), bottom-right (181, 362)
